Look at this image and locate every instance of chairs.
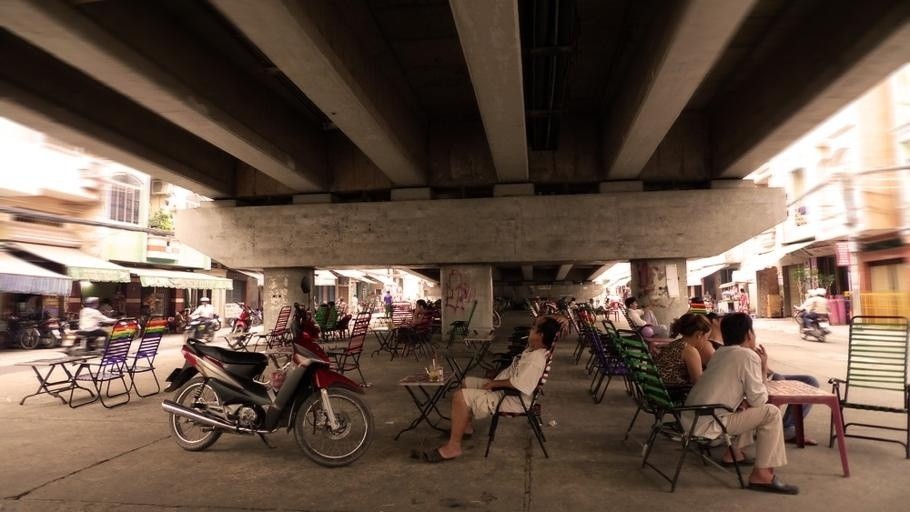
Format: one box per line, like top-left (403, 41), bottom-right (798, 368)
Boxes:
top-left (104, 315), bottom-right (169, 398)
top-left (484, 326), bottom-right (557, 460)
top-left (573, 321), bottom-right (746, 494)
top-left (525, 293), bottom-right (626, 330)
top-left (215, 299), bottom-right (443, 389)
top-left (65, 317), bottom-right (140, 409)
top-left (826, 314), bottom-right (908, 462)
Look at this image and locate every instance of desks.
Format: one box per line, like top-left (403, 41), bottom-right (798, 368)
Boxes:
top-left (12, 353), bottom-right (98, 409)
top-left (438, 341), bottom-right (485, 399)
top-left (393, 369), bottom-right (456, 442)
top-left (464, 329), bottom-right (498, 374)
top-left (741, 378), bottom-right (850, 480)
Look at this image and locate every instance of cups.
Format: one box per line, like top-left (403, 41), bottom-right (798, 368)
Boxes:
top-left (427, 366), bottom-right (443, 382)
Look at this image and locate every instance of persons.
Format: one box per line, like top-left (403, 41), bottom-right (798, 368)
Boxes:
top-left (695, 311), bottom-right (819, 445)
top-left (680, 311), bottom-right (799, 493)
top-left (740, 288), bottom-right (749, 315)
top-left (383, 290), bottom-right (394, 319)
top-left (624, 296), bottom-right (667, 336)
top-left (79, 297), bottom-right (119, 349)
top-left (313, 304), bottom-right (329, 328)
top-left (398, 299), bottom-right (432, 344)
top-left (191, 295), bottom-right (219, 330)
top-left (321, 301), bottom-right (337, 339)
top-left (422, 316), bottom-right (562, 464)
top-left (794, 290), bottom-right (815, 327)
top-left (351, 293), bottom-right (358, 315)
top-left (792, 288), bottom-right (827, 332)
top-left (335, 296), bottom-right (346, 312)
top-left (567, 297), bottom-right (578, 311)
top-left (588, 298), bottom-right (605, 314)
top-left (722, 288), bottom-right (731, 301)
top-left (605, 298), bottom-right (610, 308)
top-left (653, 312), bottom-right (714, 402)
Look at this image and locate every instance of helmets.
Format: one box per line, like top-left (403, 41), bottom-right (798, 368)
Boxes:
top-left (808, 288), bottom-right (826, 297)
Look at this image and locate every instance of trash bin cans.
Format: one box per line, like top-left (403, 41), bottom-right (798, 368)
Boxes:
top-left (827, 295), bottom-right (846, 326)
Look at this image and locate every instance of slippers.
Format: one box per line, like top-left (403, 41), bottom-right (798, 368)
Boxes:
top-left (443, 429), bottom-right (472, 440)
top-left (722, 451), bottom-right (755, 467)
top-left (424, 447), bottom-right (455, 463)
top-left (749, 473), bottom-right (799, 495)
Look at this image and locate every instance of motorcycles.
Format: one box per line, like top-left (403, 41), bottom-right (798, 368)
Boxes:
top-left (172, 308), bottom-right (252, 346)
top-left (158, 276), bottom-right (376, 467)
top-left (1, 306), bottom-right (81, 351)
top-left (790, 305), bottom-right (832, 342)
